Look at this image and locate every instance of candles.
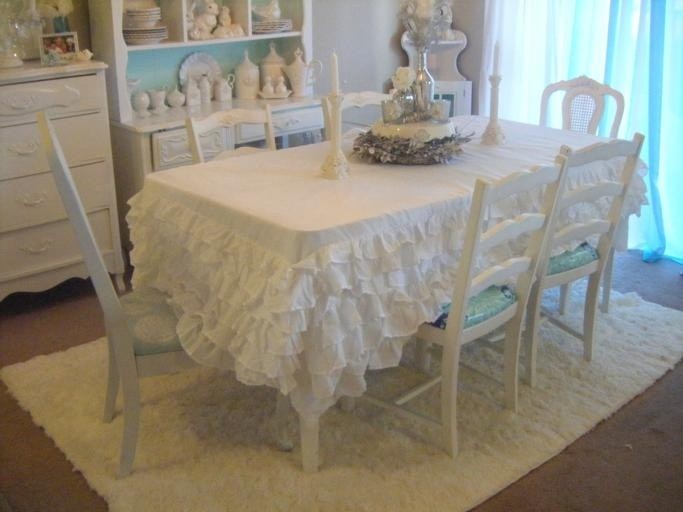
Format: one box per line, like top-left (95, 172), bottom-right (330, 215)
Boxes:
top-left (328, 48), bottom-right (340, 95)
top-left (491, 35), bottom-right (502, 78)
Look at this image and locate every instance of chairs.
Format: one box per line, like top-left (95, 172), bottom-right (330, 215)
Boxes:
top-left (321, 89), bottom-right (401, 139)
top-left (185, 104), bottom-right (276, 164)
top-left (37, 108), bottom-right (296, 479)
top-left (538, 75), bottom-right (625, 137)
top-left (482, 133), bottom-right (646, 385)
top-left (340, 154), bottom-right (566, 457)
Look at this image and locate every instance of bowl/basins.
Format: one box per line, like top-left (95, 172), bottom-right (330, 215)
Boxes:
top-left (126, 7), bottom-right (162, 27)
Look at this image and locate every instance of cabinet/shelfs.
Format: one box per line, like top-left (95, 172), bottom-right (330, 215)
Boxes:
top-left (0, 62), bottom-right (126, 304)
top-left (88, 0), bottom-right (325, 257)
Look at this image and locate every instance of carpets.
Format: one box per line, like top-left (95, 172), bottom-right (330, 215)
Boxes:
top-left (1, 278), bottom-right (683, 512)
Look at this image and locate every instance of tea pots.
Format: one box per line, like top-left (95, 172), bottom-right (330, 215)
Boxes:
top-left (280, 47), bottom-right (323, 97)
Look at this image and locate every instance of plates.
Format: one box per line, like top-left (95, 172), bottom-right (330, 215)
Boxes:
top-left (257, 90), bottom-right (293, 99)
top-left (179, 52), bottom-right (221, 100)
top-left (123, 26), bottom-right (169, 45)
